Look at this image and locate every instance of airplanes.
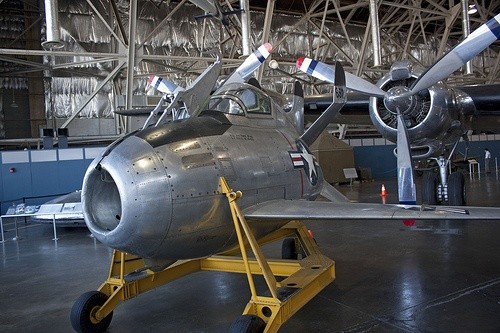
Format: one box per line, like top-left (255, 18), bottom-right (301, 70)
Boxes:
top-left (294, 13), bottom-right (500, 227)
top-left (0, 41), bottom-right (500, 333)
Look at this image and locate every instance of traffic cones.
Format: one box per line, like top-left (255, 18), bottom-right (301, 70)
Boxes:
top-left (380, 184), bottom-right (388, 195)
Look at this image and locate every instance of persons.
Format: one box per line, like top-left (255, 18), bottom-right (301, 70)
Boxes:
top-left (484, 148), bottom-right (491, 174)
top-left (237, 77), bottom-right (270, 116)
